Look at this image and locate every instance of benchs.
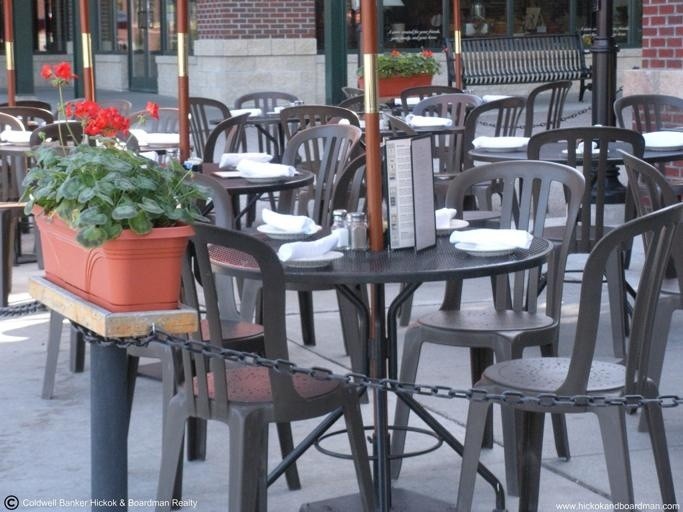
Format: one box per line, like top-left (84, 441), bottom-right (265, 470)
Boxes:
top-left (441, 30), bottom-right (619, 102)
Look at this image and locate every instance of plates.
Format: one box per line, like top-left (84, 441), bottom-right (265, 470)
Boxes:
top-left (227, 107), bottom-right (284, 119)
top-left (1, 130), bottom-right (180, 149)
top-left (239, 176), bottom-right (283, 184)
top-left (562, 148), bottom-right (608, 158)
top-left (478, 146), bottom-right (519, 153)
top-left (639, 131), bottom-right (683, 151)
top-left (433, 218), bottom-right (468, 236)
top-left (411, 124), bottom-right (447, 131)
top-left (455, 243), bottom-right (513, 257)
top-left (256, 224), bottom-right (304, 241)
top-left (286, 250), bottom-right (344, 268)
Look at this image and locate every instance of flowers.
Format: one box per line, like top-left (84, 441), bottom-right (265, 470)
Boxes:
top-left (14, 62), bottom-right (210, 246)
top-left (355, 47), bottom-right (440, 78)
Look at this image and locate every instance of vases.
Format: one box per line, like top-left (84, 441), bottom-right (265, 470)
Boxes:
top-left (357, 73), bottom-right (434, 98)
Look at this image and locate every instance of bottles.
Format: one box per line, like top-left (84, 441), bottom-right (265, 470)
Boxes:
top-left (288, 101), bottom-right (303, 107)
top-left (330, 208), bottom-right (368, 251)
top-left (167, 150), bottom-right (202, 174)
top-left (356, 112), bottom-right (385, 131)
